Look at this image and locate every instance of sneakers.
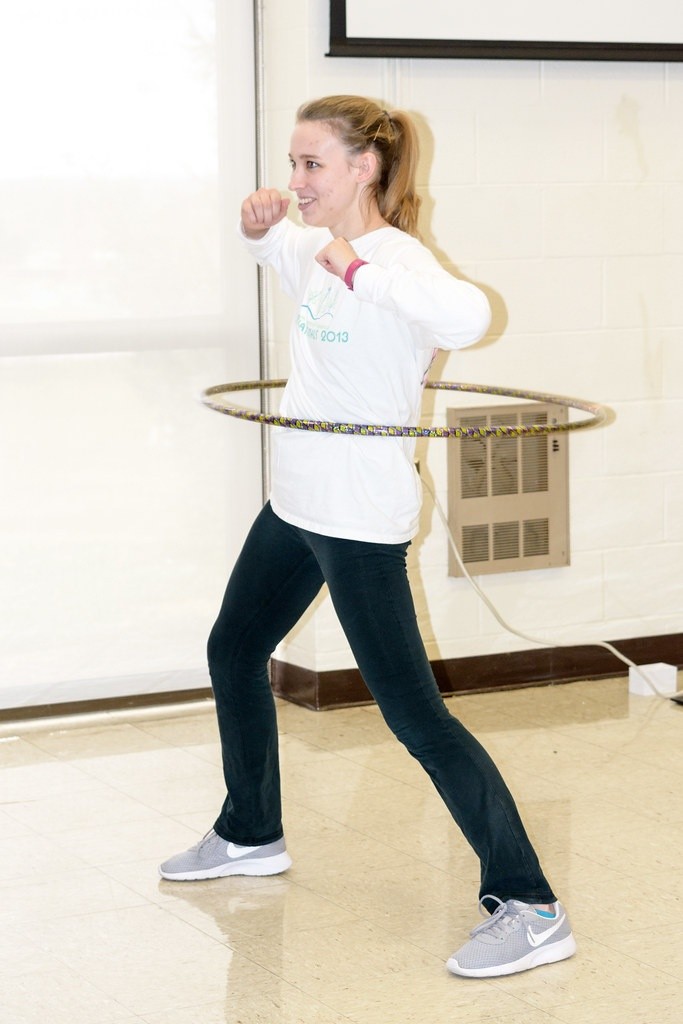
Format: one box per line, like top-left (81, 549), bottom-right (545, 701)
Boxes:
top-left (445, 894), bottom-right (576, 979)
top-left (157, 825), bottom-right (291, 883)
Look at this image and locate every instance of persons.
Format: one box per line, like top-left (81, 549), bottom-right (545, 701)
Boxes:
top-left (158, 92), bottom-right (578, 981)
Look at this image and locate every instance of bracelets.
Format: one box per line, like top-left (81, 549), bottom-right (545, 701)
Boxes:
top-left (344, 258), bottom-right (368, 291)
